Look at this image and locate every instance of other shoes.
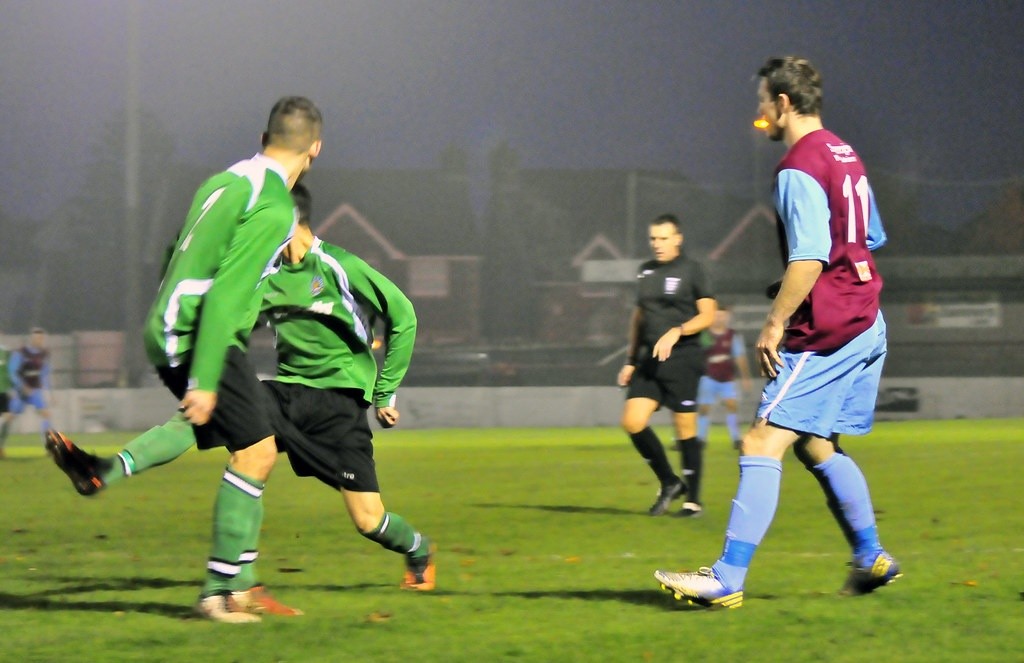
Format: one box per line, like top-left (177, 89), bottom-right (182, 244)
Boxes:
top-left (734, 439), bottom-right (742, 449)
top-left (672, 438), bottom-right (707, 450)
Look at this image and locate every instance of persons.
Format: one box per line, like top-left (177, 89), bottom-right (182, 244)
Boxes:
top-left (42, 164), bottom-right (438, 589)
top-left (616, 212), bottom-right (717, 514)
top-left (654, 56), bottom-right (903, 610)
top-left (0, 325), bottom-right (53, 457)
top-left (671, 307), bottom-right (753, 476)
top-left (141, 94), bottom-right (302, 622)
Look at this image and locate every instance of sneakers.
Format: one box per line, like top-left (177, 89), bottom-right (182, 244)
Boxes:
top-left (650, 476), bottom-right (687, 517)
top-left (227, 584), bottom-right (303, 616)
top-left (47, 428), bottom-right (113, 495)
top-left (192, 590), bottom-right (261, 623)
top-left (655, 564), bottom-right (743, 609)
top-left (836, 547), bottom-right (900, 596)
top-left (400, 540), bottom-right (438, 591)
top-left (673, 508), bottom-right (701, 519)
